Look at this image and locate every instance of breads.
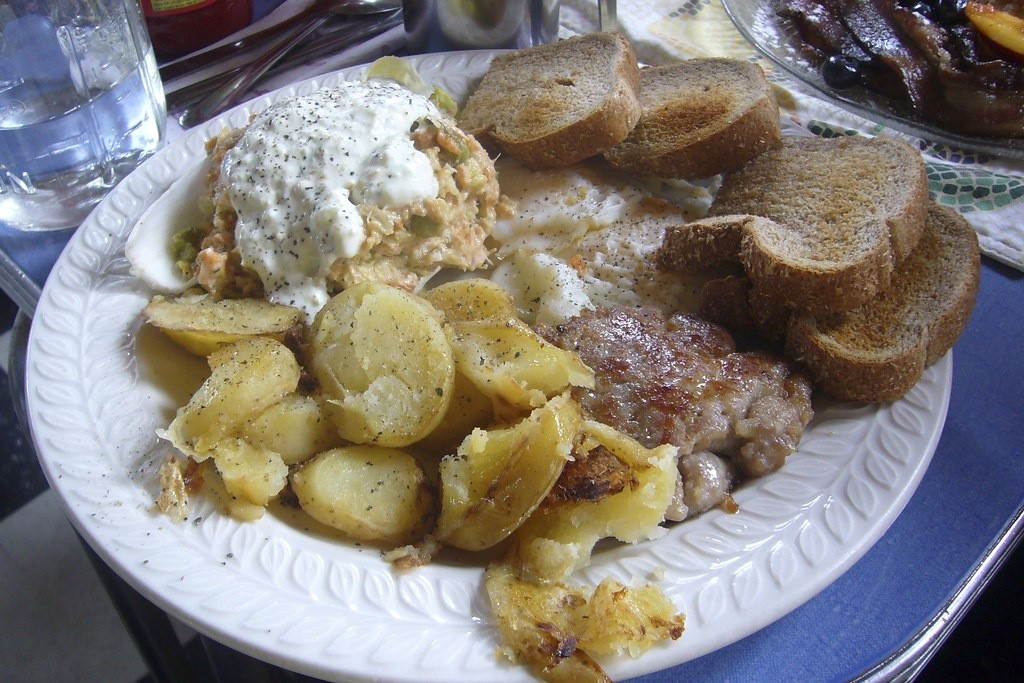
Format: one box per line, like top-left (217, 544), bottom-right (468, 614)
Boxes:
top-left (455, 32), bottom-right (981, 402)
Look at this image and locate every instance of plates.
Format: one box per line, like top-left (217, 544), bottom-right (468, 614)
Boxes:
top-left (24, 46), bottom-right (953, 683)
top-left (720, 0), bottom-right (1024, 181)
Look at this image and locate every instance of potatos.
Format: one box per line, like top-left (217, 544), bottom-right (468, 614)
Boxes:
top-left (141, 275), bottom-right (689, 682)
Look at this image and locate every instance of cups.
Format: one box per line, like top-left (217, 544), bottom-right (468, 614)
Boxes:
top-left (402, 0), bottom-right (619, 51)
top-left (0, 0), bottom-right (172, 233)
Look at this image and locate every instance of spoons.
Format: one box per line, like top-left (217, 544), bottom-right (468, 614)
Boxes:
top-left (157, 0), bottom-right (405, 131)
